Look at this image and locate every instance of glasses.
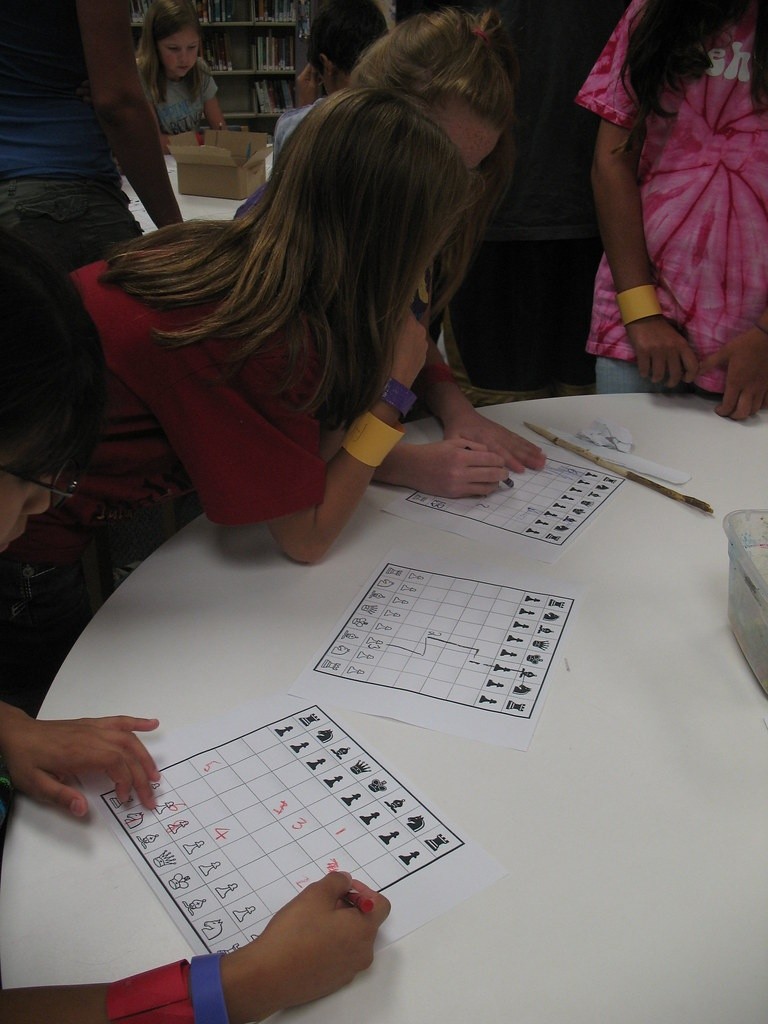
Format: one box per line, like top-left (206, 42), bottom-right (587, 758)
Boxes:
top-left (0, 462), bottom-right (81, 510)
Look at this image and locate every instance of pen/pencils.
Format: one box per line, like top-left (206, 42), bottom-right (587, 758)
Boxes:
top-left (341, 888), bottom-right (374, 913)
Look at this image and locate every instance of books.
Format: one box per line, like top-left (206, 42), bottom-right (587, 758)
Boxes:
top-left (130, 0), bottom-right (297, 113)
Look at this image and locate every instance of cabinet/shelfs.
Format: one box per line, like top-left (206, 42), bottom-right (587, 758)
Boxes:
top-left (126, 0), bottom-right (316, 134)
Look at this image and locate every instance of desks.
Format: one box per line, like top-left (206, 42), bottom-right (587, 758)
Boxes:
top-left (0, 393), bottom-right (768, 1024)
top-left (120, 141), bottom-right (273, 236)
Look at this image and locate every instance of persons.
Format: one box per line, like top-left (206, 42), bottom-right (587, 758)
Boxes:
top-left (0, 87), bottom-right (469, 718)
top-left (0, 0), bottom-right (184, 273)
top-left (136, 0), bottom-right (227, 155)
top-left (277, 0), bottom-right (768, 420)
top-left (0, 237), bottom-right (391, 1024)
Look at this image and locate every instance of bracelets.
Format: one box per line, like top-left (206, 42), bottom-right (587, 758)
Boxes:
top-left (615, 285), bottom-right (663, 326)
top-left (382, 376), bottom-right (418, 416)
top-left (105, 958), bottom-right (194, 1024)
top-left (340, 410), bottom-right (405, 467)
top-left (190, 952), bottom-right (228, 1024)
top-left (755, 323), bottom-right (768, 335)
top-left (419, 363), bottom-right (453, 385)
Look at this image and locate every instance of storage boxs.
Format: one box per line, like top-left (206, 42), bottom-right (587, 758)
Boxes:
top-left (168, 129), bottom-right (274, 200)
top-left (722, 507), bottom-right (768, 695)
